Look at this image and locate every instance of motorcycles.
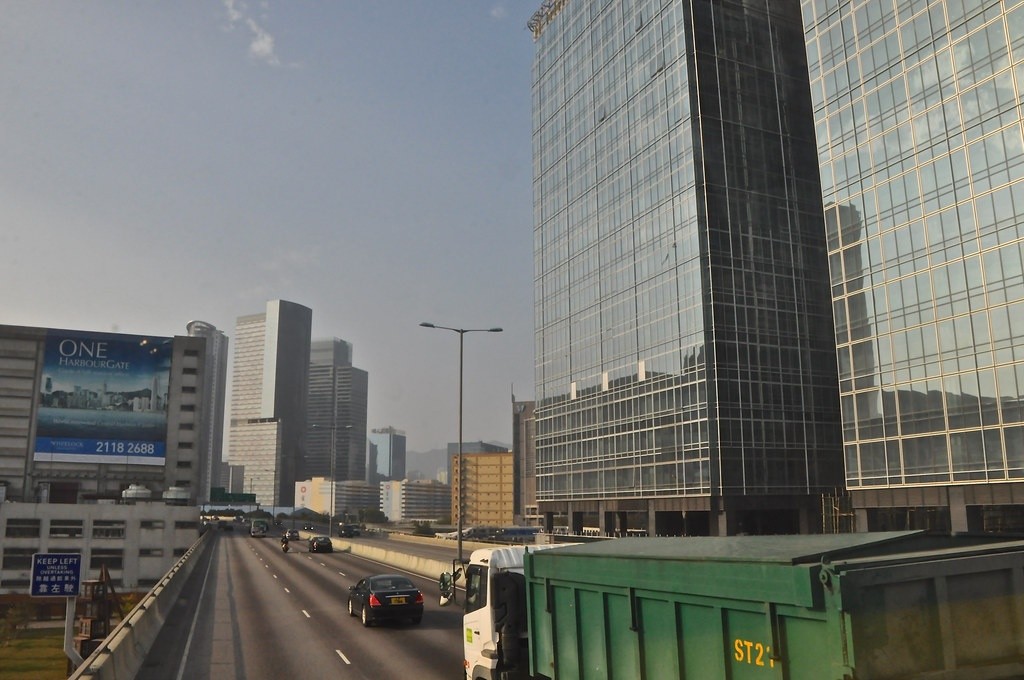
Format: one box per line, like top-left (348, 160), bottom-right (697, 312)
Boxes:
top-left (282, 544), bottom-right (289, 552)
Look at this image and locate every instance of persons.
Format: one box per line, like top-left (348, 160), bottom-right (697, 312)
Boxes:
top-left (281, 535), bottom-right (289, 546)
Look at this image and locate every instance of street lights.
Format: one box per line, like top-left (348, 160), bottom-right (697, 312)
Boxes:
top-left (243, 476), bottom-right (260, 521)
top-left (419, 322), bottom-right (504, 559)
top-left (312, 424), bottom-right (354, 537)
top-left (281, 455), bottom-right (309, 529)
top-left (265, 469), bottom-right (282, 527)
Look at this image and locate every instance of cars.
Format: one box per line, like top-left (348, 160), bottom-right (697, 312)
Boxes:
top-left (348, 574), bottom-right (424, 627)
top-left (309, 537), bottom-right (333, 553)
top-left (286, 529), bottom-right (299, 541)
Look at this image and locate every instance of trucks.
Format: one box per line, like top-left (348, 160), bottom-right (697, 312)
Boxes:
top-left (439, 530), bottom-right (1024, 680)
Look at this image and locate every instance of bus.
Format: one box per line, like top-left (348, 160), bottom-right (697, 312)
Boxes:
top-left (250, 519), bottom-right (268, 538)
